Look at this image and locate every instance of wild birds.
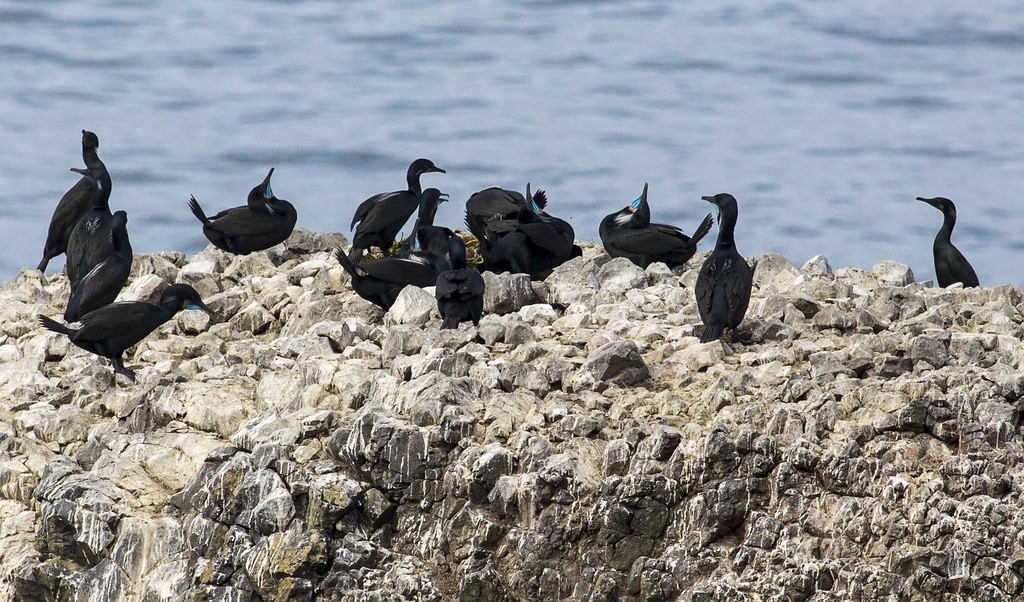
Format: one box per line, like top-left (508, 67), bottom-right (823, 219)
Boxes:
top-left (695, 193), bottom-right (755, 345)
top-left (187, 168), bottom-right (297, 256)
top-left (916, 197), bottom-right (980, 289)
top-left (334, 159), bottom-right (583, 330)
top-left (598, 182), bottom-right (714, 271)
top-left (36, 129), bottom-right (214, 383)
